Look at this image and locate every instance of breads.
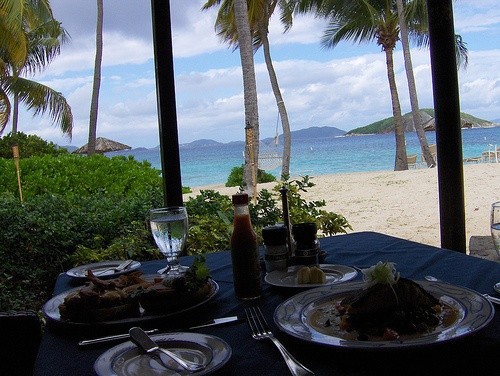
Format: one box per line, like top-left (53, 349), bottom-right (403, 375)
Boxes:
top-left (297, 266), bottom-right (326, 284)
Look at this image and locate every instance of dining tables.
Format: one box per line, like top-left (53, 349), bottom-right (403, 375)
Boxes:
top-left (35, 231), bottom-right (500, 376)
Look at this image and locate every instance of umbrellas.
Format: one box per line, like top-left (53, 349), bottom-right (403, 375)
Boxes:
top-left (423, 116), bottom-right (473, 132)
top-left (71, 137), bottom-right (132, 156)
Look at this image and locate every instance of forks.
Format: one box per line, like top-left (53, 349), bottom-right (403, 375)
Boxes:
top-left (244, 306), bottom-right (315, 376)
top-left (85, 260), bottom-right (133, 277)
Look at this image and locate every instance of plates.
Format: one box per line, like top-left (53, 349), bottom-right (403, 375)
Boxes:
top-left (42, 274), bottom-right (220, 325)
top-left (93, 332), bottom-right (232, 376)
top-left (66, 260), bottom-right (141, 280)
top-left (264, 264), bottom-right (359, 288)
top-left (272, 281), bottom-right (495, 349)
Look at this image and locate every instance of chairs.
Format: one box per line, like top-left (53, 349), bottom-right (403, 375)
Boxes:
top-left (407, 143), bottom-right (436, 169)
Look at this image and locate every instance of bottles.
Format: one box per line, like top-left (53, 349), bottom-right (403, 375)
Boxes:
top-left (231, 195), bottom-right (264, 300)
top-left (261, 225), bottom-right (289, 274)
top-left (292, 222), bottom-right (320, 257)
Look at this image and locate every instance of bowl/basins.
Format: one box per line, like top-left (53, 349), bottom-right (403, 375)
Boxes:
top-left (290, 250), bottom-right (327, 264)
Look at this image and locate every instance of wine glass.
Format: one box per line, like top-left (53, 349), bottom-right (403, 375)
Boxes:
top-left (150, 206), bottom-right (190, 275)
top-left (489, 201), bottom-right (500, 293)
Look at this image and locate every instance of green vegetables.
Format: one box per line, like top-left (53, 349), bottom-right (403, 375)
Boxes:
top-left (359, 259), bottom-right (400, 304)
top-left (178, 253), bottom-right (212, 291)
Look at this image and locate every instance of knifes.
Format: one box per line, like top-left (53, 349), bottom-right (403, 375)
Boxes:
top-left (77, 314), bottom-right (260, 346)
top-left (425, 275), bottom-right (500, 305)
